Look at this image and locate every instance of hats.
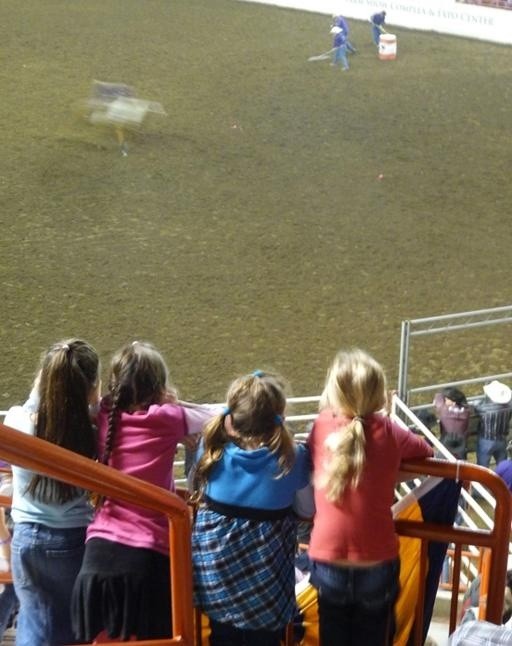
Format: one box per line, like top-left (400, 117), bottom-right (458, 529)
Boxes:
top-left (442, 388), bottom-right (469, 408)
top-left (483, 380), bottom-right (512, 404)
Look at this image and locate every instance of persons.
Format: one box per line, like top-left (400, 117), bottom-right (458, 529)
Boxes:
top-left (188, 371), bottom-right (308, 646)
top-left (4, 337), bottom-right (101, 646)
top-left (329, 27), bottom-right (349, 72)
top-left (433, 388), bottom-right (468, 459)
top-left (1, 459), bottom-right (17, 641)
top-left (496, 444), bottom-right (512, 491)
top-left (331, 15), bottom-right (349, 39)
top-left (307, 349), bottom-right (435, 645)
top-left (473, 380), bottom-right (512, 467)
top-left (69, 341), bottom-right (215, 639)
top-left (368, 10), bottom-right (386, 45)
